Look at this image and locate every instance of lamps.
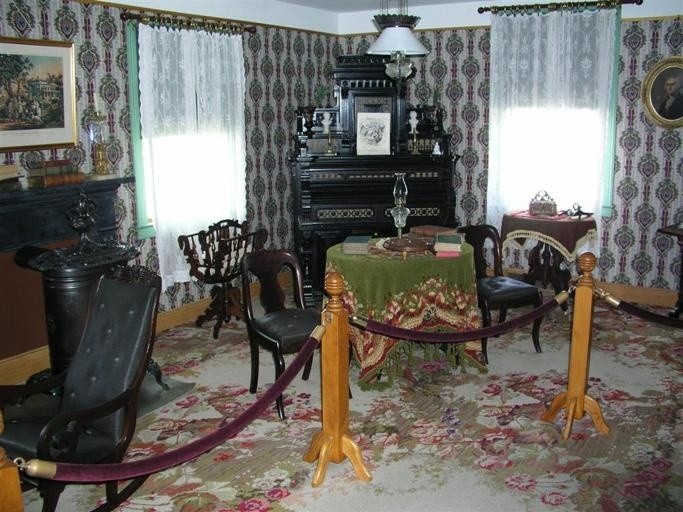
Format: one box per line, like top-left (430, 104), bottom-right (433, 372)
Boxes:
top-left (365, 0), bottom-right (431, 79)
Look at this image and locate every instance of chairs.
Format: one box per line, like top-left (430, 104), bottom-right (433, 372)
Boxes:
top-left (178, 218), bottom-right (269, 339)
top-left (0, 265), bottom-right (162, 511)
top-left (240, 249), bottom-right (353, 421)
top-left (457, 225), bottom-right (545, 365)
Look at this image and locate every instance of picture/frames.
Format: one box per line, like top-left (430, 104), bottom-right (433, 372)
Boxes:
top-left (0, 37), bottom-right (77, 153)
top-left (641, 56), bottom-right (683, 129)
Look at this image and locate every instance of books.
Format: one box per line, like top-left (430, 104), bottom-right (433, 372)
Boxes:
top-left (0, 158), bottom-right (85, 195)
top-left (342, 236), bottom-right (372, 255)
top-left (409, 225), bottom-right (466, 258)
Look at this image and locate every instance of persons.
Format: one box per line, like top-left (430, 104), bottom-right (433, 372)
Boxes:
top-left (658, 76), bottom-right (683, 119)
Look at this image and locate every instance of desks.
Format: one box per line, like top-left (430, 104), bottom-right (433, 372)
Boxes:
top-left (321, 237), bottom-right (490, 392)
top-left (0, 174), bottom-right (136, 359)
top-left (501, 209), bottom-right (597, 312)
